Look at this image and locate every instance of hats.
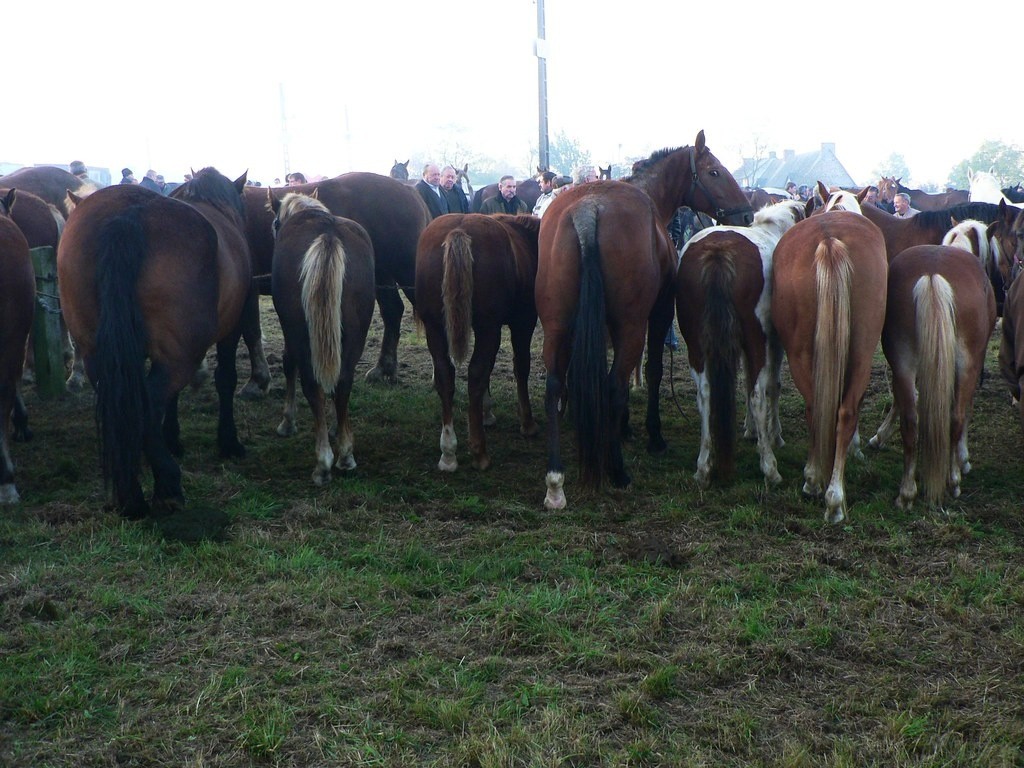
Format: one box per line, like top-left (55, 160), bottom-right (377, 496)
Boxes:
top-left (122, 168), bottom-right (132, 177)
top-left (157, 175), bottom-right (163, 182)
top-left (70, 161), bottom-right (85, 174)
top-left (552, 176), bottom-right (573, 189)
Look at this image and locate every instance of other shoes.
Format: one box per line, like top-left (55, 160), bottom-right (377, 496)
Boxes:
top-left (663, 342), bottom-right (677, 352)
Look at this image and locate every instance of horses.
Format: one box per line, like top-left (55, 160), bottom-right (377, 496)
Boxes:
top-left (0, 128), bottom-right (1024, 525)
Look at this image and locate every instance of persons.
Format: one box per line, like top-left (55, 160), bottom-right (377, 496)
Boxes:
top-left (782, 182), bottom-right (809, 202)
top-left (864, 186), bottom-right (922, 219)
top-left (665, 208), bottom-right (682, 350)
top-left (68, 160), bottom-right (307, 196)
top-left (415, 163), bottom-right (599, 218)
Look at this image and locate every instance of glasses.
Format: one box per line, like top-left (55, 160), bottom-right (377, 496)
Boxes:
top-left (289, 180), bottom-right (299, 183)
top-left (184, 180), bottom-right (188, 182)
top-left (586, 175), bottom-right (599, 180)
